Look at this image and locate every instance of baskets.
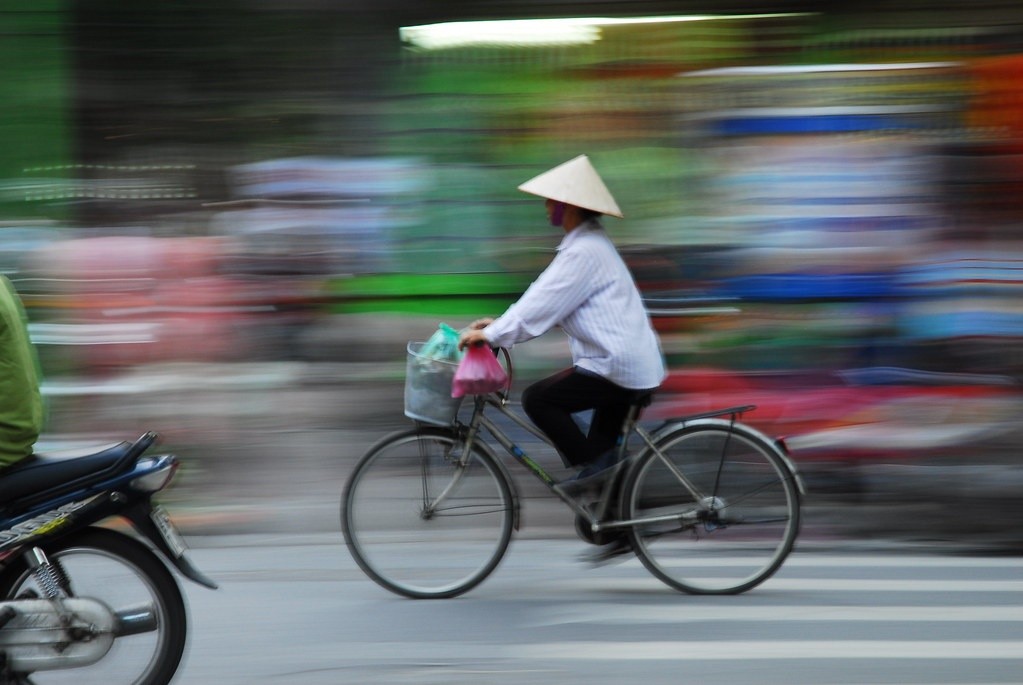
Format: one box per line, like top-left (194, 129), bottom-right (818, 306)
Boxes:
top-left (405, 340), bottom-right (467, 429)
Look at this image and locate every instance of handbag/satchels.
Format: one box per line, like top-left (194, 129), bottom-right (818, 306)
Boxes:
top-left (451, 338), bottom-right (508, 397)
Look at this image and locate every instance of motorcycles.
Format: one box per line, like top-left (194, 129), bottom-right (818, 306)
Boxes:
top-left (0, 427), bottom-right (217, 684)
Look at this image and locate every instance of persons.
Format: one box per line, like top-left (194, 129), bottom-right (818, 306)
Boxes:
top-left (0, 278), bottom-right (44, 472)
top-left (457, 153), bottom-right (666, 566)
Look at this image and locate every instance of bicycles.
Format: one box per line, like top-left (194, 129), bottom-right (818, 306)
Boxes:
top-left (339, 326), bottom-right (807, 601)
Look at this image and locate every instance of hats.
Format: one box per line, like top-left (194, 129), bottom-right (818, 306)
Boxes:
top-left (517, 153), bottom-right (622, 218)
